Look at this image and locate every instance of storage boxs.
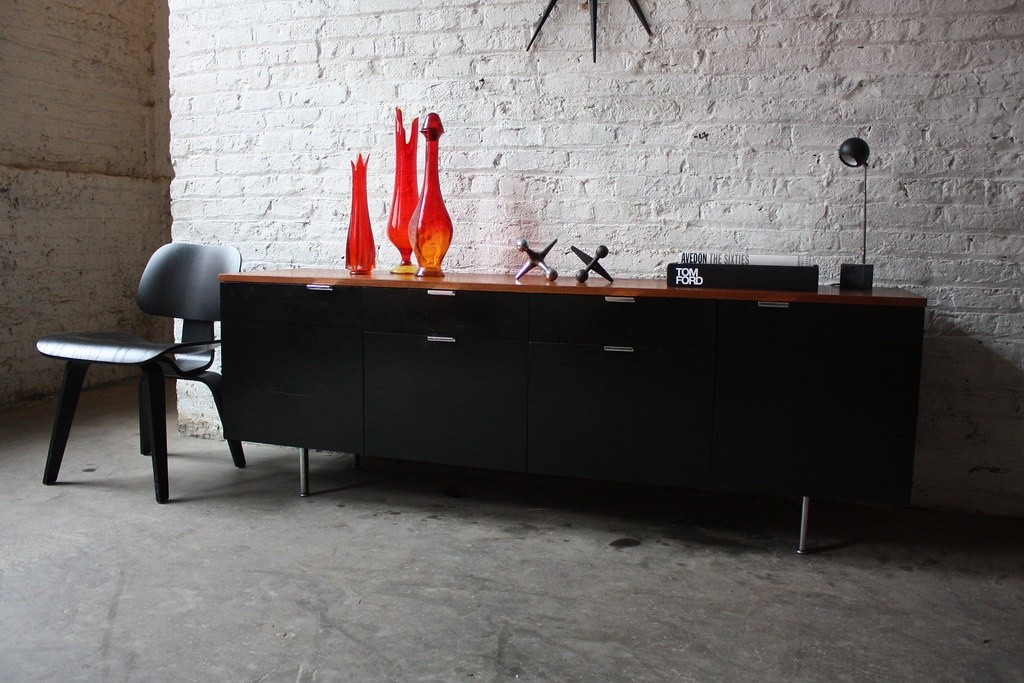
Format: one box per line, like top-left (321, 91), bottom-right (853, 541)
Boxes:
top-left (667, 263), bottom-right (819, 292)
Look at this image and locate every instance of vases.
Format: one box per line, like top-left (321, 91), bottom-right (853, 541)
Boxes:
top-left (344, 153), bottom-right (377, 276)
top-left (387, 107), bottom-right (420, 274)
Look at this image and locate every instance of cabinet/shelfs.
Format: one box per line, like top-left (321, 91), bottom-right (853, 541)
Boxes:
top-left (220, 273), bottom-right (928, 554)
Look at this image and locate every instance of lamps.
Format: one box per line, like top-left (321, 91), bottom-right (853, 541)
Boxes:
top-left (839, 137), bottom-right (874, 290)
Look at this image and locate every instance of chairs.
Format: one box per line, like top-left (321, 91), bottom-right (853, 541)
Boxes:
top-left (36, 242), bottom-right (247, 504)
